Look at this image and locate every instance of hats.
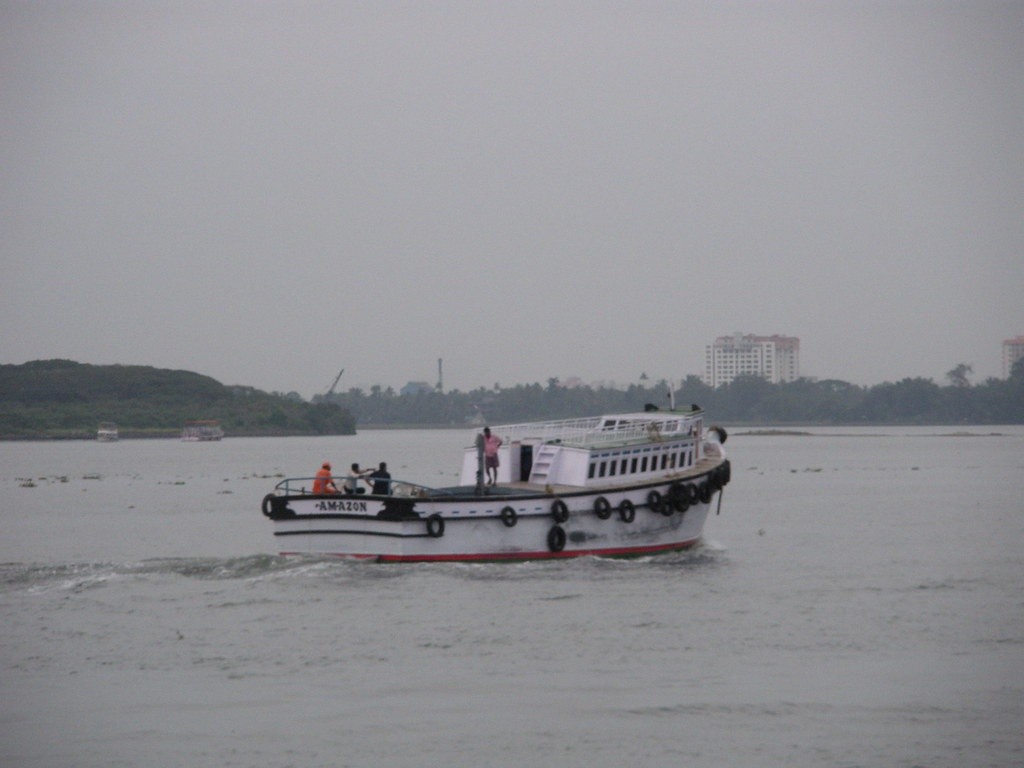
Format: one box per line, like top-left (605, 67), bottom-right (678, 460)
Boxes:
top-left (322, 462), bottom-right (329, 466)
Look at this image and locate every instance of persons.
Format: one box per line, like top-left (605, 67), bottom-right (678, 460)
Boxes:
top-left (481, 427), bottom-right (502, 485)
top-left (312, 462), bottom-right (392, 495)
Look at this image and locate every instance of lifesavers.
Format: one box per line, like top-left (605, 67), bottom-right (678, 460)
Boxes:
top-left (262, 494), bottom-right (280, 517)
top-left (619, 499), bottom-right (634, 523)
top-left (427, 514), bottom-right (445, 538)
top-left (500, 506), bottom-right (518, 528)
top-left (551, 500), bottom-right (569, 523)
top-left (658, 497), bottom-right (673, 516)
top-left (648, 490), bottom-right (660, 512)
top-left (700, 460), bottom-right (731, 504)
top-left (547, 526), bottom-right (566, 552)
top-left (594, 496), bottom-right (612, 519)
top-left (687, 483), bottom-right (699, 505)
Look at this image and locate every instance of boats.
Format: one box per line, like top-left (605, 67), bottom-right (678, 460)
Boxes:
top-left (97, 420), bottom-right (120, 442)
top-left (261, 402), bottom-right (731, 563)
top-left (180, 419), bottom-right (225, 442)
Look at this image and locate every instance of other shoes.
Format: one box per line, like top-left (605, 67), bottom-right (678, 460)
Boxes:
top-left (492, 484), bottom-right (496, 486)
top-left (488, 480), bottom-right (492, 484)
